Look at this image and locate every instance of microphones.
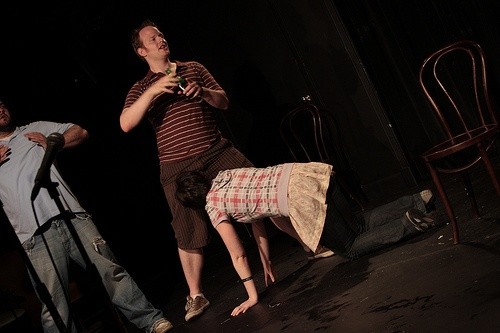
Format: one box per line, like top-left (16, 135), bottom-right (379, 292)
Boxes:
top-left (31, 132), bottom-right (65, 203)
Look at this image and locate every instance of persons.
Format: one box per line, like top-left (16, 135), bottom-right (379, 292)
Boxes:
top-left (0, 102), bottom-right (172, 333)
top-left (172, 161), bottom-right (450, 316)
top-left (118, 23), bottom-right (335, 321)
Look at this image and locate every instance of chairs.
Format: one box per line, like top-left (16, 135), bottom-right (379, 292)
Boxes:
top-left (418, 41), bottom-right (500, 244)
top-left (279, 103), bottom-right (363, 245)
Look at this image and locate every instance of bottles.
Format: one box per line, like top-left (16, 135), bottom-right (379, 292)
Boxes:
top-left (164, 68), bottom-right (196, 99)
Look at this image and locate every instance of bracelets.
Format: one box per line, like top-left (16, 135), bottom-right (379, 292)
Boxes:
top-left (241, 275), bottom-right (253, 282)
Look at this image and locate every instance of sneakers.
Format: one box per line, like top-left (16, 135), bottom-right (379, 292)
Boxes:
top-left (185, 294), bottom-right (209, 321)
top-left (153, 317), bottom-right (172, 333)
top-left (420, 188), bottom-right (436, 210)
top-left (306, 245), bottom-right (335, 260)
top-left (405, 208), bottom-right (450, 232)
